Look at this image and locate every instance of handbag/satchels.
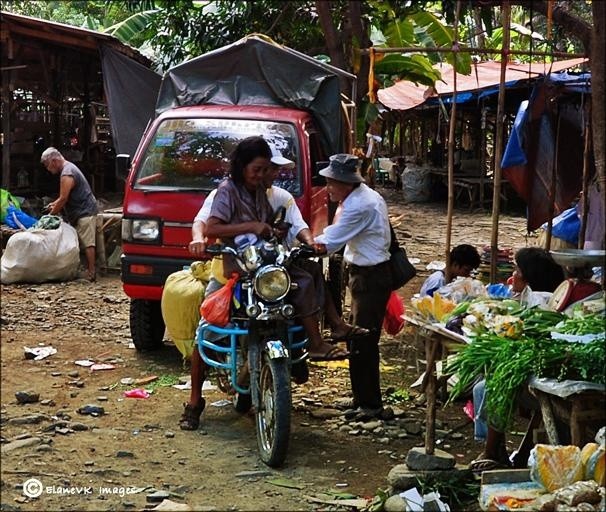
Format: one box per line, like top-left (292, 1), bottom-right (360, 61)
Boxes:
top-left (389, 247), bottom-right (415, 291)
top-left (261, 223), bottom-right (292, 263)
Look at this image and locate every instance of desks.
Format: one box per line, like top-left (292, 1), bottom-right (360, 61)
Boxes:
top-left (417, 330), bottom-right (605, 467)
top-left (450, 180), bottom-right (509, 213)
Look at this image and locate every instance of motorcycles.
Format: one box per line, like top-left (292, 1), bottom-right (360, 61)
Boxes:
top-left (188, 234), bottom-right (327, 469)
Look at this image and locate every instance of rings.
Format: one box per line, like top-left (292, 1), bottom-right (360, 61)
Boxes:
top-left (191, 248), bottom-right (196, 251)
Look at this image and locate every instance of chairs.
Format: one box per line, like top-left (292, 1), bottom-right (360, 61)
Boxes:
top-left (167, 155), bottom-right (215, 188)
top-left (374, 156), bottom-right (389, 187)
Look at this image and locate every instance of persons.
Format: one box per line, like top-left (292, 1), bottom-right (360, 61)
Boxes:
top-left (179, 147), bottom-right (308, 430)
top-left (41, 147), bottom-right (100, 284)
top-left (562, 256), bottom-right (602, 310)
top-left (309, 152), bottom-right (392, 419)
top-left (420, 244), bottom-right (481, 355)
top-left (467, 247), bottom-right (565, 469)
top-left (206, 137), bottom-right (371, 363)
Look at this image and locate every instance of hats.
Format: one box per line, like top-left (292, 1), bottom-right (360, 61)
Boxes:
top-left (268, 143), bottom-right (294, 168)
top-left (319, 154), bottom-right (366, 184)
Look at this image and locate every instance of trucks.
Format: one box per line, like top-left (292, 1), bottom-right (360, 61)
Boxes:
top-left (116, 101), bottom-right (348, 354)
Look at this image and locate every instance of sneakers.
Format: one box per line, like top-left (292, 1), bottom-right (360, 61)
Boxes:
top-left (335, 396), bottom-right (382, 421)
top-left (180, 396), bottom-right (205, 431)
top-left (287, 344), bottom-right (308, 384)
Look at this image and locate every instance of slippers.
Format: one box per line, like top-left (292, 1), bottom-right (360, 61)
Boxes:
top-left (331, 325), bottom-right (370, 342)
top-left (310, 347), bottom-right (352, 361)
top-left (469, 458), bottom-right (513, 471)
